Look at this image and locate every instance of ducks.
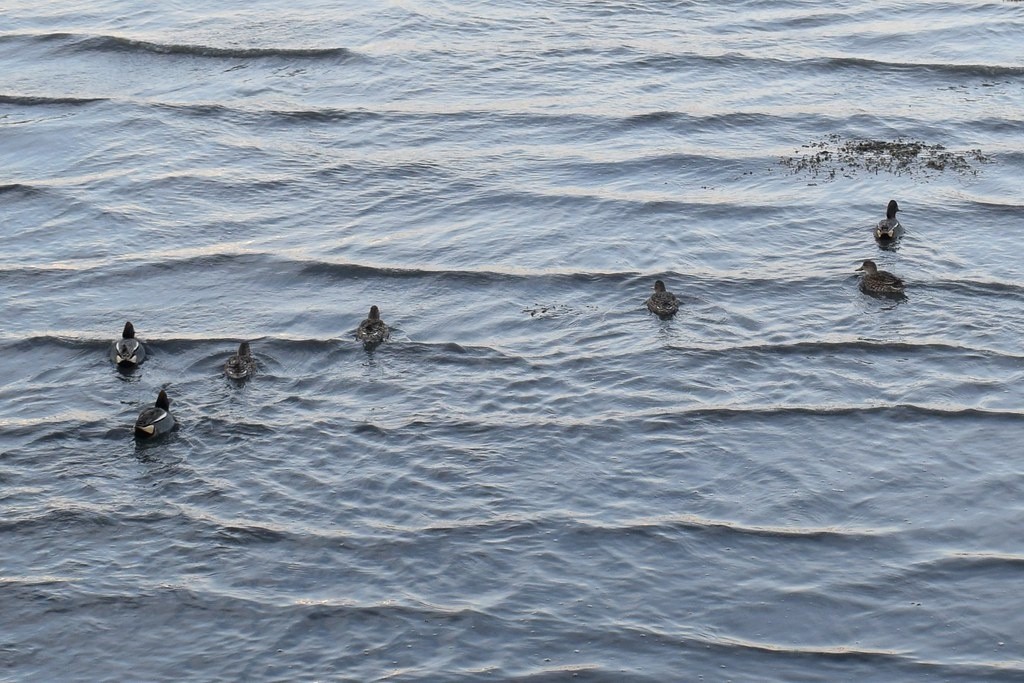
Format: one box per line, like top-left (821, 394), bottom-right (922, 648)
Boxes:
top-left (132, 389), bottom-right (175, 441)
top-left (647, 280), bottom-right (679, 316)
top-left (357, 305), bottom-right (390, 349)
top-left (854, 261), bottom-right (908, 297)
top-left (109, 322), bottom-right (145, 369)
top-left (874, 200), bottom-right (903, 240)
top-left (224, 340), bottom-right (256, 382)
